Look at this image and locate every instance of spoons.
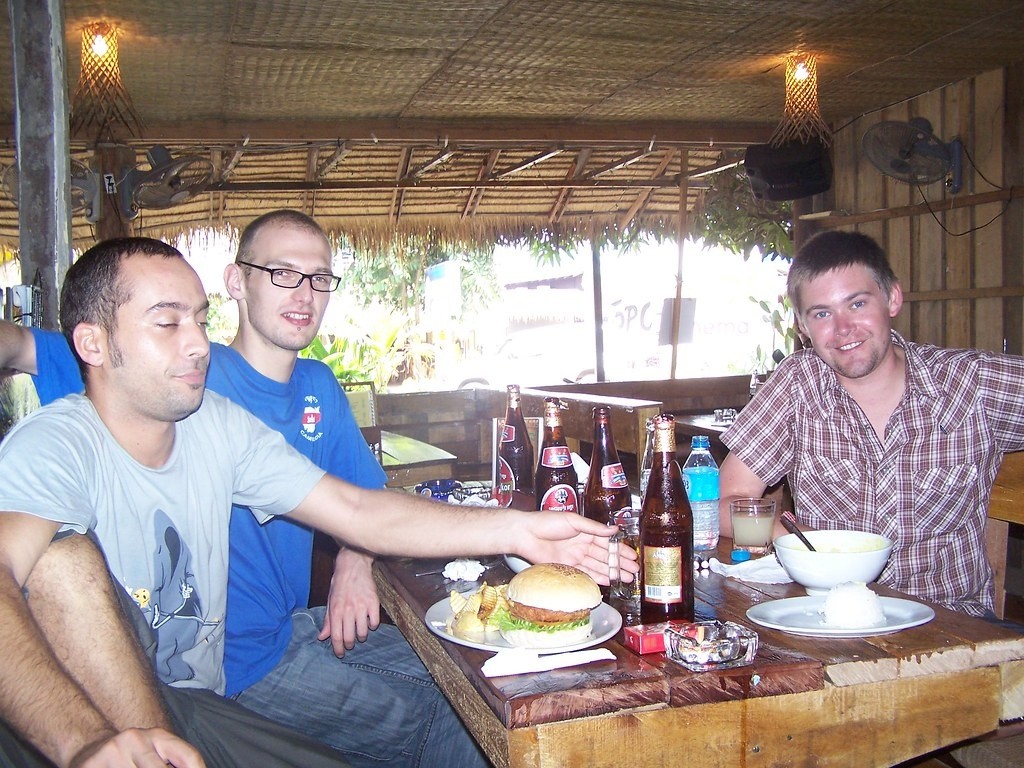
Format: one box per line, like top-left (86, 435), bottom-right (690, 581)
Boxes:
top-left (504, 554), bottom-right (531, 574)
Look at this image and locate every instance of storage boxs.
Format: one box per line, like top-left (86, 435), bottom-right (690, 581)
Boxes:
top-left (624, 617), bottom-right (698, 655)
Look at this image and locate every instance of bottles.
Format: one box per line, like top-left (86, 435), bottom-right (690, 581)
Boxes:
top-left (639, 414), bottom-right (694, 625)
top-left (535, 397), bottom-right (580, 514)
top-left (584, 406), bottom-right (632, 524)
top-left (640, 419), bottom-right (656, 508)
top-left (681, 436), bottom-right (720, 551)
top-left (749, 370), bottom-right (760, 400)
top-left (499, 385), bottom-right (536, 511)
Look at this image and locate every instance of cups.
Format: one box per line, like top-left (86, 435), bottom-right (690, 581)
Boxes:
top-left (421, 479), bottom-right (459, 501)
top-left (730, 498), bottom-right (776, 554)
top-left (714, 410), bottom-right (723, 422)
top-left (608, 509), bottom-right (641, 616)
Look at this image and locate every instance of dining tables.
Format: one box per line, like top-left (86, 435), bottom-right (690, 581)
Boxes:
top-left (371, 528), bottom-right (1023, 768)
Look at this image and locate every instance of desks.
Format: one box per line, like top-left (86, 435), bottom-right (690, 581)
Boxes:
top-left (380, 428), bottom-right (458, 488)
top-left (672, 413), bottom-right (797, 515)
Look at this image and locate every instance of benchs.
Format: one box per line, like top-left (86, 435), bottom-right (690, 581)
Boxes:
top-left (451, 386), bottom-right (708, 483)
top-left (951, 450), bottom-right (1024, 768)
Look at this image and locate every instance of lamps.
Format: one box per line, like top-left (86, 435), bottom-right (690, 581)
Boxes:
top-left (766, 54), bottom-right (838, 147)
top-left (68, 20), bottom-right (148, 146)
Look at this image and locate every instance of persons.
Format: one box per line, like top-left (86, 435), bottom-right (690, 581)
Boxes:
top-left (720, 231), bottom-right (1024, 635)
top-left (0, 236), bottom-right (640, 768)
top-left (0, 211), bottom-right (494, 768)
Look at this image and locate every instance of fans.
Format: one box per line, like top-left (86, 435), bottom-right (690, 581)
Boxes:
top-left (121, 142), bottom-right (215, 220)
top-left (861, 112), bottom-right (964, 194)
top-left (2, 159), bottom-right (101, 224)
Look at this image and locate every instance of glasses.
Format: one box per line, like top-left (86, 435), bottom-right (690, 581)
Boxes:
top-left (238, 260), bottom-right (342, 293)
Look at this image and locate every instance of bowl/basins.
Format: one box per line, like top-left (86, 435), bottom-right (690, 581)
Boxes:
top-left (773, 530), bottom-right (893, 596)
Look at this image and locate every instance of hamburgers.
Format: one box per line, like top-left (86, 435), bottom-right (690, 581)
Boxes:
top-left (498, 563), bottom-right (603, 648)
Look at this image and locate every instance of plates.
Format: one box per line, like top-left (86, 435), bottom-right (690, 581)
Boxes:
top-left (424, 590), bottom-right (622, 654)
top-left (746, 596), bottom-right (935, 638)
